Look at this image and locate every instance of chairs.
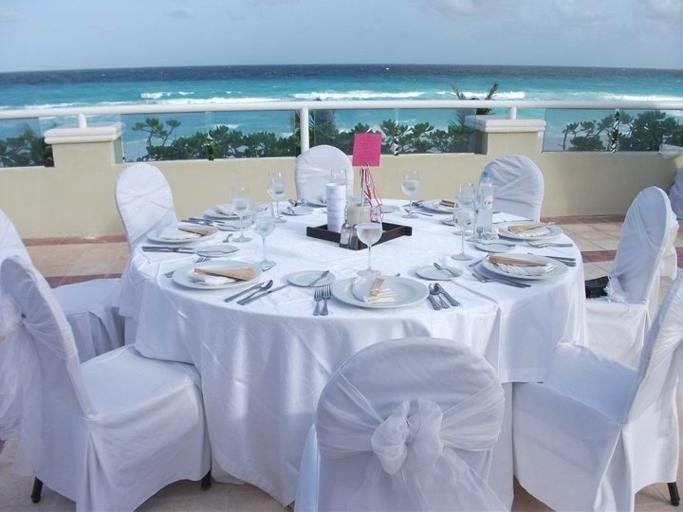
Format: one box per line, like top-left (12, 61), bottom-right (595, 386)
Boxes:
top-left (292, 336), bottom-right (506, 512)
top-left (513, 272), bottom-right (683, 512)
top-left (0, 254), bottom-right (213, 512)
top-left (113, 163), bottom-right (178, 254)
top-left (0, 209), bottom-right (126, 362)
top-left (585, 185), bottom-right (679, 368)
top-left (293, 144), bottom-right (354, 202)
top-left (479, 154), bottom-right (544, 222)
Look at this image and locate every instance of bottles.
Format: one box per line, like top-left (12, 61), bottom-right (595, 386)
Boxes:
top-left (349, 223), bottom-right (360, 250)
top-left (476, 170), bottom-right (494, 241)
top-left (338, 223), bottom-right (349, 246)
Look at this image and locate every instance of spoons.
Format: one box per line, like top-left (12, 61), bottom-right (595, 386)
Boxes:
top-left (428, 282), bottom-right (449, 311)
top-left (236, 279), bottom-right (272, 304)
top-left (222, 233), bottom-right (232, 243)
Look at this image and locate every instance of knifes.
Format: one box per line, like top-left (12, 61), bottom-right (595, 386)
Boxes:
top-left (402, 199), bottom-right (422, 207)
top-left (242, 284), bottom-right (289, 305)
top-left (178, 217), bottom-right (224, 226)
top-left (306, 270), bottom-right (330, 287)
top-left (225, 281), bottom-right (262, 303)
top-left (435, 283), bottom-right (459, 307)
top-left (141, 246), bottom-right (194, 254)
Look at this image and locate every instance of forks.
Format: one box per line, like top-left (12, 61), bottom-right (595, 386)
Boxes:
top-left (165, 255), bottom-right (210, 279)
top-left (471, 269), bottom-right (530, 288)
top-left (476, 241), bottom-right (515, 247)
top-left (319, 283), bottom-right (330, 315)
top-left (433, 262), bottom-right (458, 277)
top-left (314, 286), bottom-right (321, 316)
top-left (526, 241), bottom-right (573, 248)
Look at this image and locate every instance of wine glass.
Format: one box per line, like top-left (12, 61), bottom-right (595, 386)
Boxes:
top-left (449, 180), bottom-right (477, 261)
top-left (250, 201), bottom-right (278, 268)
top-left (353, 211), bottom-right (385, 277)
top-left (230, 186), bottom-right (251, 242)
top-left (264, 168), bottom-right (289, 224)
top-left (400, 168), bottom-right (419, 219)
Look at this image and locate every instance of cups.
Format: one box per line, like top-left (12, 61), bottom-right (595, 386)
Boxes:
top-left (325, 183), bottom-right (345, 233)
top-left (330, 167), bottom-right (346, 185)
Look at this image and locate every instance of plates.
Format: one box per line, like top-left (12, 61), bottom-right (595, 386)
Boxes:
top-left (205, 206), bottom-right (247, 219)
top-left (329, 274), bottom-right (427, 310)
top-left (493, 220), bottom-right (562, 240)
top-left (421, 198), bottom-right (454, 214)
top-left (481, 252), bottom-right (567, 282)
top-left (282, 206), bottom-right (315, 216)
top-left (286, 269), bottom-right (334, 288)
top-left (415, 265), bottom-right (463, 281)
top-left (193, 243), bottom-right (236, 258)
top-left (441, 217), bottom-right (455, 226)
top-left (170, 259), bottom-right (259, 290)
top-left (307, 197), bottom-right (328, 206)
top-left (146, 221), bottom-right (217, 243)
